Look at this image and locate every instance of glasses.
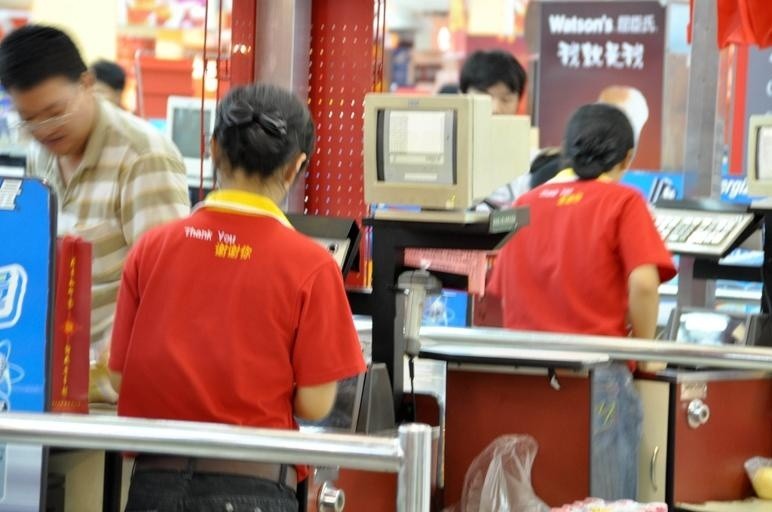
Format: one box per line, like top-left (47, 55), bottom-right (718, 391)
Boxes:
top-left (16, 82), bottom-right (86, 132)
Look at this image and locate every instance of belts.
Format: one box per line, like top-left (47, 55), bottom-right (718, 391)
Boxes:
top-left (131, 454), bottom-right (299, 493)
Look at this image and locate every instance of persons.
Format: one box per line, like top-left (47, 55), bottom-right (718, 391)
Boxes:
top-left (0, 25), bottom-right (191, 512)
top-left (460, 49), bottom-right (525, 114)
top-left (483, 103), bottom-right (680, 375)
top-left (109, 84), bottom-right (368, 511)
top-left (89, 60), bottom-right (125, 109)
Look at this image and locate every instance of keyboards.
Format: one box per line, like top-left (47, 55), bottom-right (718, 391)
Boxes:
top-left (651, 208), bottom-right (754, 255)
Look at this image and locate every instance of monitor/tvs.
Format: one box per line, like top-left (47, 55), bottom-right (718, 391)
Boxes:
top-left (747, 115), bottom-right (772, 210)
top-left (166, 96), bottom-right (216, 178)
top-left (365, 93), bottom-right (539, 224)
